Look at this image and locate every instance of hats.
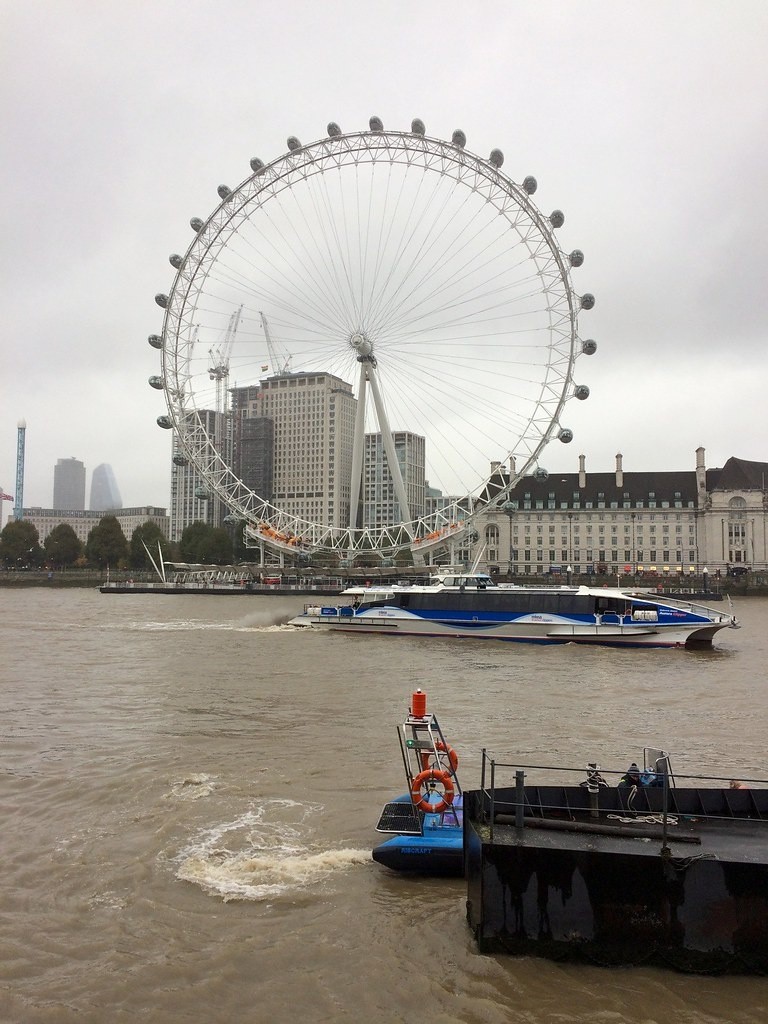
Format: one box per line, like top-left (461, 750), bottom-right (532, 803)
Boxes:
top-left (627, 763), bottom-right (640, 776)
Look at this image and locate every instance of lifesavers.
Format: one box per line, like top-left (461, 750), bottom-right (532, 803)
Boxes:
top-left (421, 741), bottom-right (458, 778)
top-left (412, 770), bottom-right (455, 813)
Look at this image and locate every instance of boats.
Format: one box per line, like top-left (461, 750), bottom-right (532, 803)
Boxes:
top-left (286, 573), bottom-right (741, 651)
top-left (370, 689), bottom-right (464, 876)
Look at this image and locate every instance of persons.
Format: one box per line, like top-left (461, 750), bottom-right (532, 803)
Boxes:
top-left (616, 763), bottom-right (643, 787)
top-left (353, 596), bottom-right (359, 609)
top-left (730, 780), bottom-right (754, 790)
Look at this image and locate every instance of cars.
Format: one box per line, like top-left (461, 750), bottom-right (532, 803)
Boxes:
top-left (729, 567), bottom-right (748, 576)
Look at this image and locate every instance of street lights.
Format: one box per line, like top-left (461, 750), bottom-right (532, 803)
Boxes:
top-left (509, 515), bottom-right (513, 572)
top-left (763, 519), bottom-right (767, 562)
top-left (751, 518), bottom-right (755, 561)
top-left (631, 512), bottom-right (635, 561)
top-left (694, 510), bottom-right (699, 576)
top-left (721, 518), bottom-right (725, 560)
top-left (568, 513), bottom-right (572, 568)
top-left (680, 560), bottom-right (684, 574)
